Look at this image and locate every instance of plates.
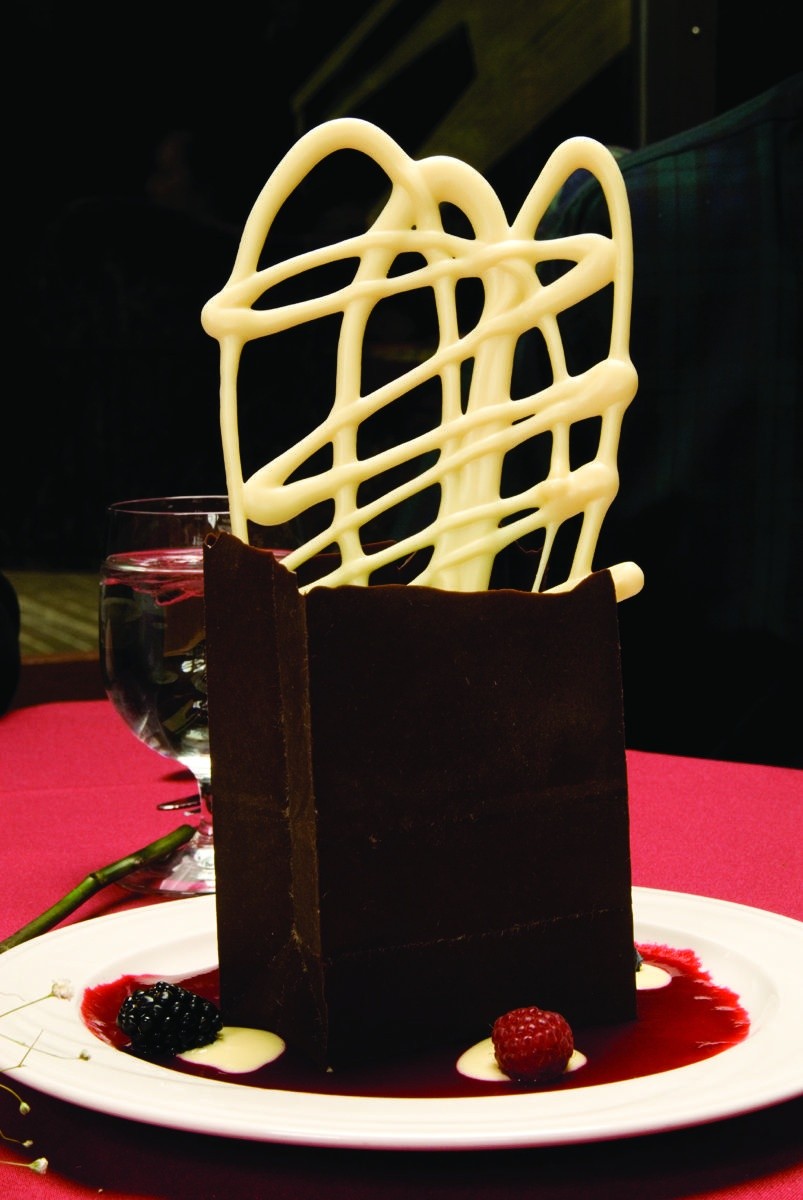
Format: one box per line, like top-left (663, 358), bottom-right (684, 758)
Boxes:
top-left (0, 887), bottom-right (803, 1152)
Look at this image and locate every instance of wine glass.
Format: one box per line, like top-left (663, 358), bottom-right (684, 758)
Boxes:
top-left (96, 494), bottom-right (297, 901)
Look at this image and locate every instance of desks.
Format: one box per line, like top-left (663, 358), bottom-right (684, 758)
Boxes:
top-left (0, 701), bottom-right (803, 1200)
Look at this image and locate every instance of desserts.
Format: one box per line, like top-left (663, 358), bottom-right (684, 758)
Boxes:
top-left (197, 124), bottom-right (660, 1067)
